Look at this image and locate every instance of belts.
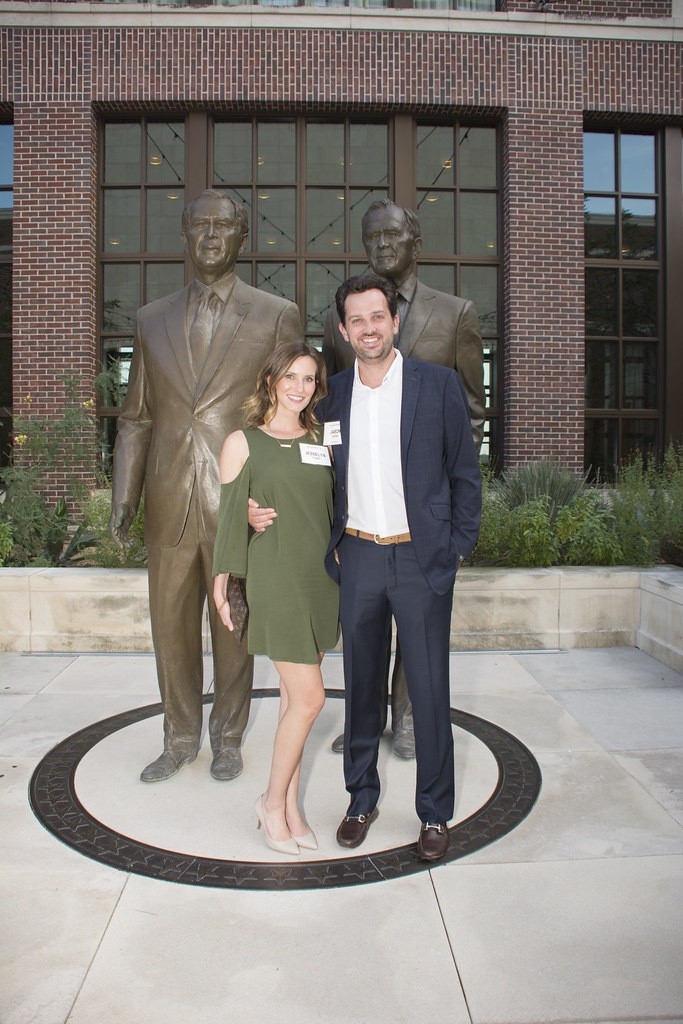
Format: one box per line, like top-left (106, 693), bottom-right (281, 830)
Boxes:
top-left (344, 527), bottom-right (412, 546)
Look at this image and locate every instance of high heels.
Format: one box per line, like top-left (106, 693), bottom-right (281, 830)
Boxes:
top-left (254, 793), bottom-right (300, 855)
top-left (292, 831), bottom-right (318, 850)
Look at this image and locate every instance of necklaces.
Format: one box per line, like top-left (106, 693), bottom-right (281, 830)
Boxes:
top-left (270, 431), bottom-right (297, 449)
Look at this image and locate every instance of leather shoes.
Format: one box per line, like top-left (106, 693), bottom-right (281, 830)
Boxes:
top-left (336, 805), bottom-right (376, 848)
top-left (418, 822), bottom-right (450, 860)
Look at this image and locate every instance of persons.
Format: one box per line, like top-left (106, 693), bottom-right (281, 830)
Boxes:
top-left (212, 341), bottom-right (340, 854)
top-left (248, 273), bottom-right (484, 860)
top-left (323, 199), bottom-right (487, 762)
top-left (109, 187), bottom-right (304, 782)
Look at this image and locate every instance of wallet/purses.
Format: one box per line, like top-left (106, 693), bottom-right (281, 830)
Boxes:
top-left (226, 577), bottom-right (248, 645)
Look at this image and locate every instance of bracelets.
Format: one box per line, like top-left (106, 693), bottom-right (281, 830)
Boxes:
top-left (217, 598), bottom-right (229, 614)
